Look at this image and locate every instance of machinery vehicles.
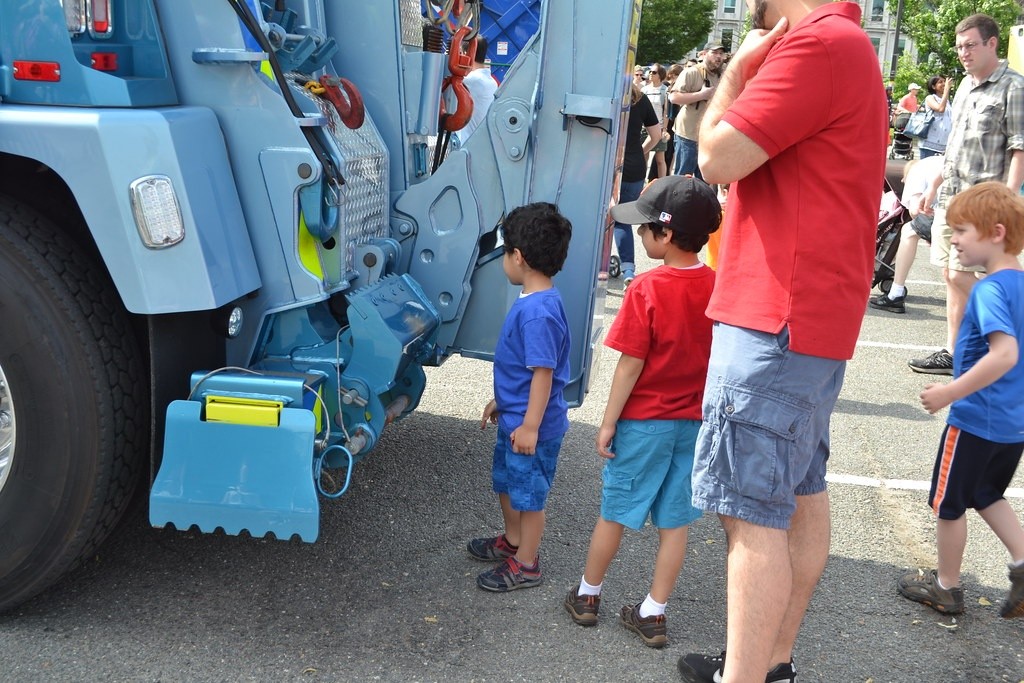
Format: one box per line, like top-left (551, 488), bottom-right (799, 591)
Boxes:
top-left (0, 0), bottom-right (642, 606)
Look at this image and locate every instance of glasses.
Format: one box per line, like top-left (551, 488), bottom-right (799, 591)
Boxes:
top-left (634, 74), bottom-right (643, 77)
top-left (649, 71), bottom-right (658, 75)
top-left (953, 38), bottom-right (990, 54)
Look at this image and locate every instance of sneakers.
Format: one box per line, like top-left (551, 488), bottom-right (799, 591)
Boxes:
top-left (908, 348), bottom-right (953, 377)
top-left (676, 651), bottom-right (799, 683)
top-left (477, 554), bottom-right (542, 592)
top-left (869, 294), bottom-right (905, 313)
top-left (466, 533), bottom-right (519, 561)
top-left (999, 562), bottom-right (1024, 619)
top-left (897, 568), bottom-right (965, 614)
top-left (619, 602), bottom-right (667, 647)
top-left (564, 582), bottom-right (601, 625)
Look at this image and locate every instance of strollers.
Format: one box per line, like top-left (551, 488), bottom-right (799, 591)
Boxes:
top-left (856, 158), bottom-right (914, 296)
top-left (889, 112), bottom-right (914, 160)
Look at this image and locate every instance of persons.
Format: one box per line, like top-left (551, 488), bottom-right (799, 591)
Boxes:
top-left (868, 73), bottom-right (966, 314)
top-left (908, 13), bottom-right (1024, 374)
top-left (675, 0), bottom-right (889, 683)
top-left (466, 201), bottom-right (572, 592)
top-left (897, 181), bottom-right (1024, 619)
top-left (565, 174), bottom-right (722, 648)
top-left (614, 42), bottom-right (734, 291)
top-left (444, 33), bottom-right (503, 260)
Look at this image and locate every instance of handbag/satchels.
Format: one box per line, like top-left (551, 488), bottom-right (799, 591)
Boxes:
top-left (903, 95), bottom-right (938, 138)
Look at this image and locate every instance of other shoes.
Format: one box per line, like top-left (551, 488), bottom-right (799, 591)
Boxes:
top-left (622, 269), bottom-right (636, 292)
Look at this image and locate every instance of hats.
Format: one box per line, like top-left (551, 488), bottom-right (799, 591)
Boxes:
top-left (703, 42), bottom-right (725, 51)
top-left (908, 83), bottom-right (921, 91)
top-left (609, 176), bottom-right (718, 235)
